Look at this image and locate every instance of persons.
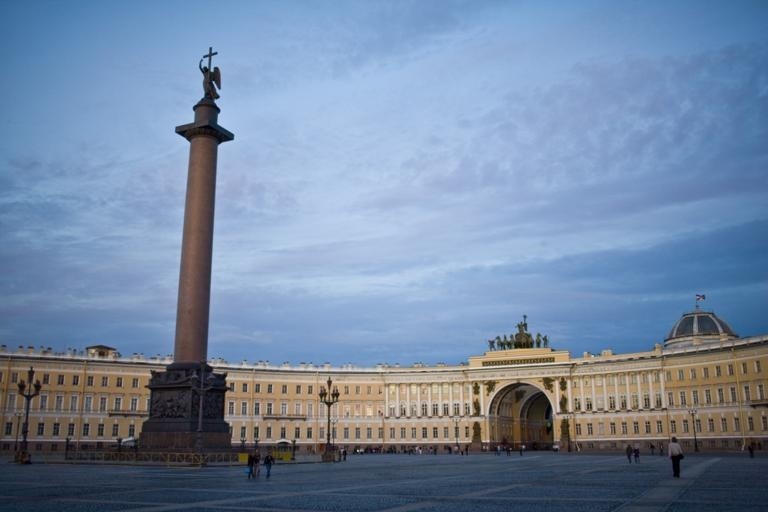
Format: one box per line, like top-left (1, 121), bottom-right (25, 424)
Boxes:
top-left (262, 450), bottom-right (276, 478)
top-left (668, 436), bottom-right (684, 479)
top-left (352, 443), bottom-right (528, 456)
top-left (625, 444), bottom-right (633, 464)
top-left (342, 449), bottom-right (346, 461)
top-left (515, 322), bottom-right (525, 333)
top-left (246, 453), bottom-right (256, 480)
top-left (575, 440), bottom-right (582, 456)
top-left (553, 441), bottom-right (559, 456)
top-left (633, 448), bottom-right (641, 464)
top-left (487, 338), bottom-right (497, 351)
top-left (747, 442), bottom-right (755, 458)
top-left (198, 57), bottom-right (221, 102)
top-left (648, 442), bottom-right (655, 456)
top-left (252, 447), bottom-right (261, 477)
top-left (658, 441), bottom-right (664, 455)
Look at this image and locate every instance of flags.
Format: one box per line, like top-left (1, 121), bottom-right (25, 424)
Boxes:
top-left (696, 294), bottom-right (705, 302)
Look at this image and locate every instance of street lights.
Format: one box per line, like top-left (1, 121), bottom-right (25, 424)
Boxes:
top-left (688, 407), bottom-right (698, 452)
top-left (319, 376), bottom-right (340, 462)
top-left (13, 366), bottom-right (42, 465)
